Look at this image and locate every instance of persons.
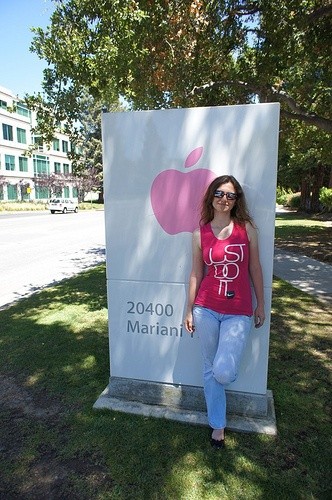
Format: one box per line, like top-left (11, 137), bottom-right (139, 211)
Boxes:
top-left (183, 174), bottom-right (265, 450)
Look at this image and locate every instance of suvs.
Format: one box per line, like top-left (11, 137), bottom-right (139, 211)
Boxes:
top-left (48, 198), bottom-right (80, 214)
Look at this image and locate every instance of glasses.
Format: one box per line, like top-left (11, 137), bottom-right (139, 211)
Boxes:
top-left (213, 190), bottom-right (237, 200)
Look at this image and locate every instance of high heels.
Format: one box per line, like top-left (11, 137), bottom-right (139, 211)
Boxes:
top-left (211, 432), bottom-right (224, 450)
